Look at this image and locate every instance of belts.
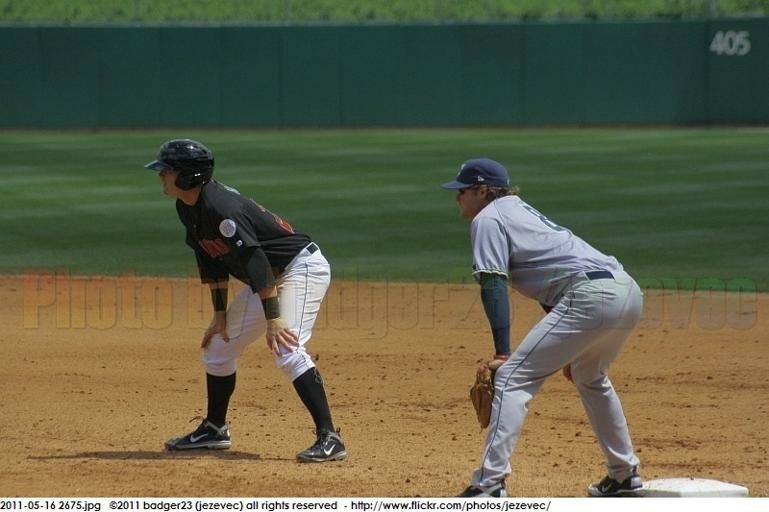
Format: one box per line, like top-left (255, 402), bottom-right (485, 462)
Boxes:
top-left (585, 268), bottom-right (614, 280)
top-left (305, 242), bottom-right (319, 252)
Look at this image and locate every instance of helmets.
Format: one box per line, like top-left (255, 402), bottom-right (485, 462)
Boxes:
top-left (144, 140), bottom-right (215, 190)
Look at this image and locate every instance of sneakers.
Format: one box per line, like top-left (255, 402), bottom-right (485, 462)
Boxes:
top-left (174, 415), bottom-right (231, 449)
top-left (459, 478), bottom-right (507, 497)
top-left (588, 470), bottom-right (644, 497)
top-left (296, 427), bottom-right (346, 462)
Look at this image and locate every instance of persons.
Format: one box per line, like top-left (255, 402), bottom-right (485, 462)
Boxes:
top-left (142, 138), bottom-right (348, 461)
top-left (439, 158), bottom-right (643, 497)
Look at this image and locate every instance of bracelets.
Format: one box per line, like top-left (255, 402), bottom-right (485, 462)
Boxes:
top-left (209, 288), bottom-right (228, 311)
top-left (261, 295), bottom-right (280, 320)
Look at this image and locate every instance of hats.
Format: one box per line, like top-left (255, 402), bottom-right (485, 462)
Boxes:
top-left (441, 156), bottom-right (510, 190)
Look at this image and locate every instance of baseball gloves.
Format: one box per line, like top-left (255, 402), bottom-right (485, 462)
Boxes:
top-left (470, 355), bottom-right (511, 429)
